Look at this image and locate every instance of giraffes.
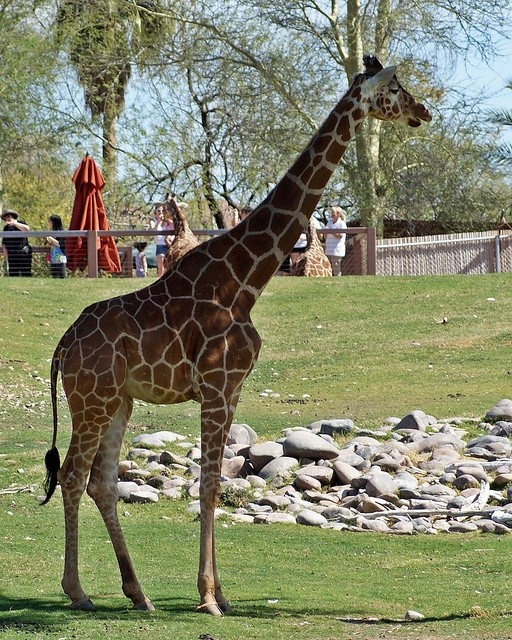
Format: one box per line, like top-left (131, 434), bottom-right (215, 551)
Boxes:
top-left (37, 54), bottom-right (432, 619)
top-left (291, 218), bottom-right (333, 277)
top-left (153, 192), bottom-right (200, 274)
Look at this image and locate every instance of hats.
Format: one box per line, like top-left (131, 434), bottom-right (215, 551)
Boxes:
top-left (1, 209), bottom-right (18, 220)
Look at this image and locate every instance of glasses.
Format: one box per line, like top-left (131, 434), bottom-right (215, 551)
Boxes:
top-left (157, 210), bottom-right (164, 212)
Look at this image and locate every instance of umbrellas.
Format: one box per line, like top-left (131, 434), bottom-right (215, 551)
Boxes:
top-left (63, 149), bottom-right (122, 273)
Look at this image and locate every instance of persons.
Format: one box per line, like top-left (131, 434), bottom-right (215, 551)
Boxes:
top-left (0, 208), bottom-right (33, 276)
top-left (239, 206), bottom-right (253, 221)
top-left (149, 202), bottom-right (174, 278)
top-left (133, 242), bottom-right (149, 278)
top-left (289, 230), bottom-right (307, 264)
top-left (323, 206), bottom-right (348, 276)
top-left (43, 214), bottom-right (68, 279)
